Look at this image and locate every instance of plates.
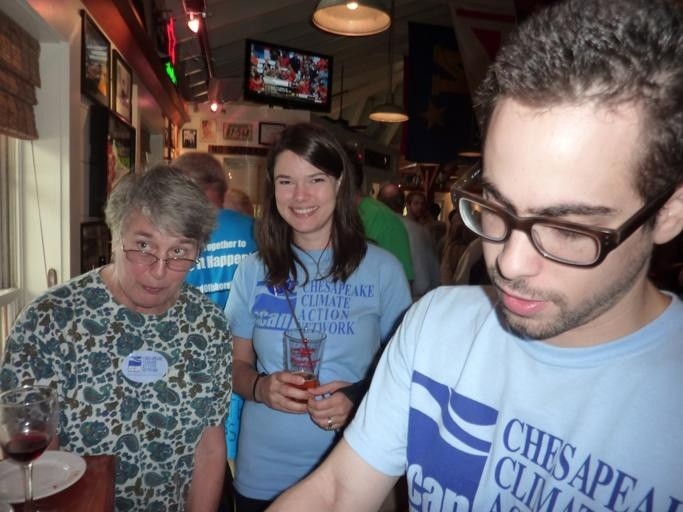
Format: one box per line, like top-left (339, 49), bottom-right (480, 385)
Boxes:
top-left (0, 449), bottom-right (87, 504)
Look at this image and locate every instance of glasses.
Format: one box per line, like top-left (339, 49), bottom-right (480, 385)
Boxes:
top-left (450, 161), bottom-right (676, 267)
top-left (122, 241), bottom-right (202, 272)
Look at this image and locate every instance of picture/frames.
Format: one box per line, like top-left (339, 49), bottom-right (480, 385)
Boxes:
top-left (112, 49), bottom-right (133, 124)
top-left (182, 128), bottom-right (197, 150)
top-left (80, 9), bottom-right (111, 110)
top-left (258, 123), bottom-right (287, 145)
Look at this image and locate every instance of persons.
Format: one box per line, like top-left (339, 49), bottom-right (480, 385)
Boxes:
top-left (345, 141), bottom-right (414, 294)
top-left (263, 0), bottom-right (683, 511)
top-left (170, 154), bottom-right (260, 503)
top-left (224, 189), bottom-right (254, 218)
top-left (1, 166), bottom-right (234, 512)
top-left (250, 47), bottom-right (329, 103)
top-left (376, 184), bottom-right (491, 301)
top-left (223, 121), bottom-right (413, 512)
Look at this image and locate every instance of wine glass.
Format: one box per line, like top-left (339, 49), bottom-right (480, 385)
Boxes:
top-left (0, 385), bottom-right (59, 512)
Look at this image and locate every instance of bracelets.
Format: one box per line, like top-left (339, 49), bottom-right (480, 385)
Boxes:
top-left (253, 374), bottom-right (265, 404)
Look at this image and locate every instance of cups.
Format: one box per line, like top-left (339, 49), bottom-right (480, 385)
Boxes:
top-left (284, 328), bottom-right (327, 405)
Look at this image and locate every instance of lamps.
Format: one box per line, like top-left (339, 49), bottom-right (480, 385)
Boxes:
top-left (312, 0), bottom-right (410, 123)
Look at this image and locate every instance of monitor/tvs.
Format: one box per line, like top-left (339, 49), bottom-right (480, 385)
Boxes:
top-left (243, 38), bottom-right (334, 113)
